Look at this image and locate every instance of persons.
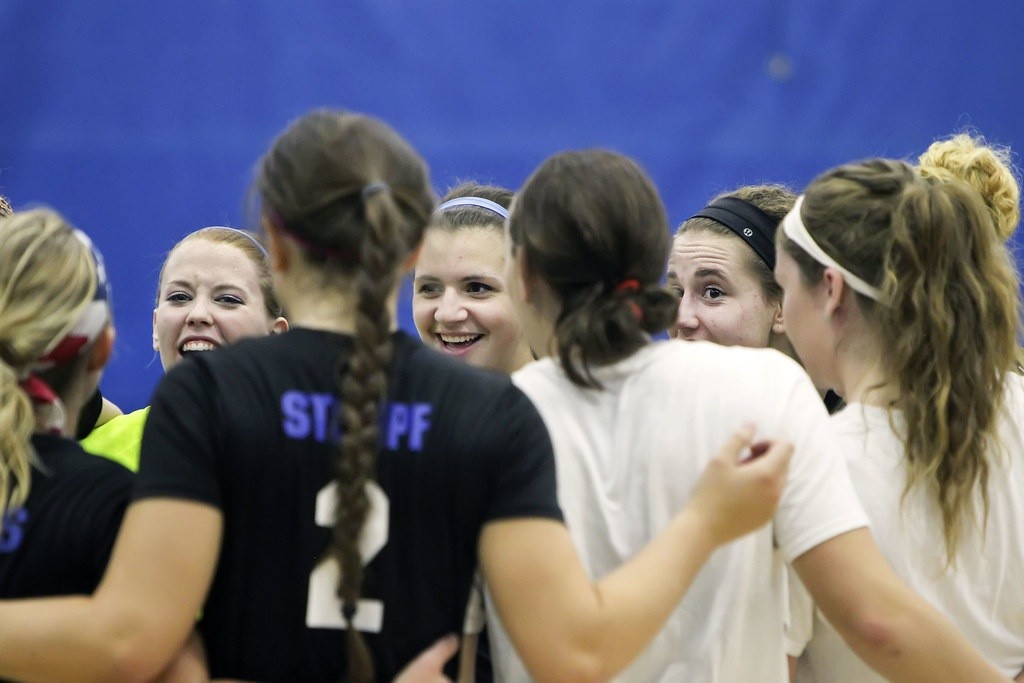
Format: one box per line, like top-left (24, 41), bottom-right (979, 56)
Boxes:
top-left (773, 135), bottom-right (1024, 683)
top-left (0, 208), bottom-right (208, 683)
top-left (411, 184), bottom-right (538, 377)
top-left (664, 183), bottom-right (849, 415)
top-left (77, 227), bottom-right (292, 473)
top-left (0, 109), bottom-right (795, 683)
top-left (458, 150), bottom-right (1012, 683)
